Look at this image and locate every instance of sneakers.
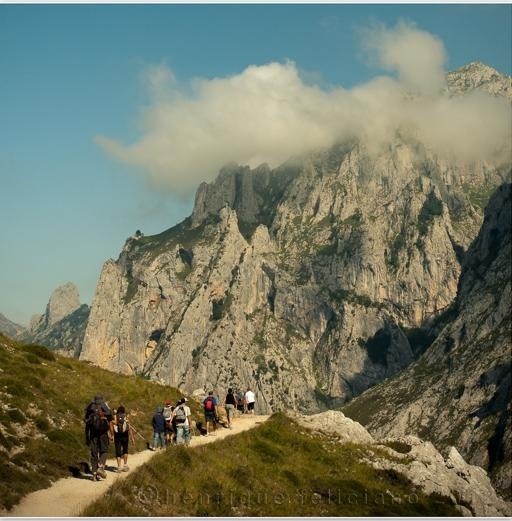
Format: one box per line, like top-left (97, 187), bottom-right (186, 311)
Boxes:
top-left (93, 474), bottom-right (99, 481)
top-left (97, 467), bottom-right (107, 478)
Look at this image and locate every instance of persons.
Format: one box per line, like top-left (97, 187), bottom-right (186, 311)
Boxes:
top-left (221, 388), bottom-right (238, 428)
top-left (152, 397), bottom-right (194, 452)
top-left (237, 386), bottom-right (255, 414)
top-left (108, 404), bottom-right (134, 472)
top-left (84, 392), bottom-right (114, 482)
top-left (203, 391), bottom-right (220, 438)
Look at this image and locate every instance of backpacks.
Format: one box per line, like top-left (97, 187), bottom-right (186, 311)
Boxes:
top-left (113, 414), bottom-right (127, 433)
top-left (175, 406), bottom-right (186, 423)
top-left (205, 397), bottom-right (213, 411)
top-left (163, 406), bottom-right (172, 420)
top-left (90, 412), bottom-right (110, 433)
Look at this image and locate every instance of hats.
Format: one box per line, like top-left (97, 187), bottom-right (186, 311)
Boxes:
top-left (181, 398), bottom-right (188, 402)
